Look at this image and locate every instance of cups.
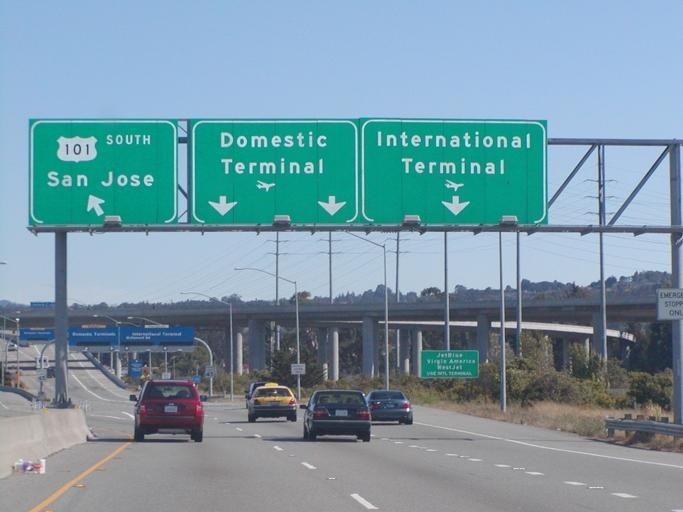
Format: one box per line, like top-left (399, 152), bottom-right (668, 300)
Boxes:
top-left (39, 459), bottom-right (46, 474)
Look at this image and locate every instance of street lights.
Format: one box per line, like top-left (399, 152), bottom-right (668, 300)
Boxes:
top-left (233, 266), bottom-right (302, 400)
top-left (117, 321), bottom-right (153, 381)
top-left (127, 316), bottom-right (168, 380)
top-left (91, 314), bottom-right (119, 327)
top-left (179, 291), bottom-right (234, 401)
top-left (3, 309), bottom-right (25, 372)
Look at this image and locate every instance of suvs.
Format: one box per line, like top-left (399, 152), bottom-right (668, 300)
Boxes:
top-left (128, 379), bottom-right (204, 442)
top-left (242, 382), bottom-right (265, 408)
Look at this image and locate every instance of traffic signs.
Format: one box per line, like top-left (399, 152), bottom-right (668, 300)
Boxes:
top-left (420, 350), bottom-right (480, 378)
top-left (29, 300), bottom-right (57, 309)
top-left (359, 118), bottom-right (546, 225)
top-left (19, 327), bottom-right (57, 345)
top-left (68, 326), bottom-right (120, 346)
top-left (189, 118), bottom-right (360, 230)
top-left (27, 117), bottom-right (180, 226)
top-left (121, 326), bottom-right (195, 346)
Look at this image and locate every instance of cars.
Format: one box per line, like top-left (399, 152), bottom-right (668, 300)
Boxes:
top-left (364, 390), bottom-right (415, 425)
top-left (7, 343), bottom-right (19, 352)
top-left (46, 365), bottom-right (56, 378)
top-left (245, 383), bottom-right (300, 422)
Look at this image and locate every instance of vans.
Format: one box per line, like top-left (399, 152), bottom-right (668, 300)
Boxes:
top-left (298, 388), bottom-right (372, 443)
